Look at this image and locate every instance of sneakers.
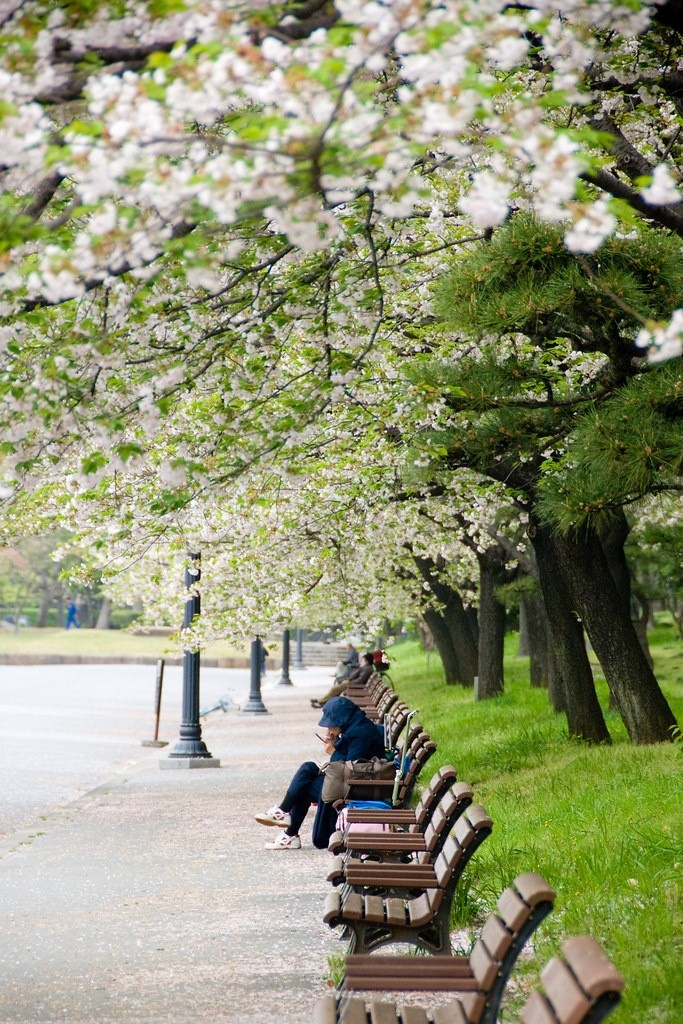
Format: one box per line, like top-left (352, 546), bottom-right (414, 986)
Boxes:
top-left (264, 831), bottom-right (301, 849)
top-left (255, 805), bottom-right (291, 827)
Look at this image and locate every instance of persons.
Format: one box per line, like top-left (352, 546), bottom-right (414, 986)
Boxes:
top-left (254, 697), bottom-right (385, 848)
top-left (260, 642), bottom-right (269, 675)
top-left (330, 643), bottom-right (358, 677)
top-left (311, 654), bottom-right (374, 708)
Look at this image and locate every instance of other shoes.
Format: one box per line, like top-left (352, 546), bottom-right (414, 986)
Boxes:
top-left (311, 702), bottom-right (322, 708)
top-left (310, 698), bottom-right (318, 703)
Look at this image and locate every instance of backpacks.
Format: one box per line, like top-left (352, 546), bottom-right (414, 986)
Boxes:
top-left (335, 801), bottom-right (414, 863)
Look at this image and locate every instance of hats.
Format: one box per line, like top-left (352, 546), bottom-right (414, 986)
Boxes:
top-left (362, 653), bottom-right (373, 665)
top-left (318, 696), bottom-right (360, 727)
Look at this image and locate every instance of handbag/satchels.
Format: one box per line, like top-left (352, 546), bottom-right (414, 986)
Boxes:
top-left (319, 756), bottom-right (396, 803)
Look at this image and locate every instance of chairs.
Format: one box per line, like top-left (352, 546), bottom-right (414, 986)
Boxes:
top-left (314, 672), bottom-right (623, 1024)
